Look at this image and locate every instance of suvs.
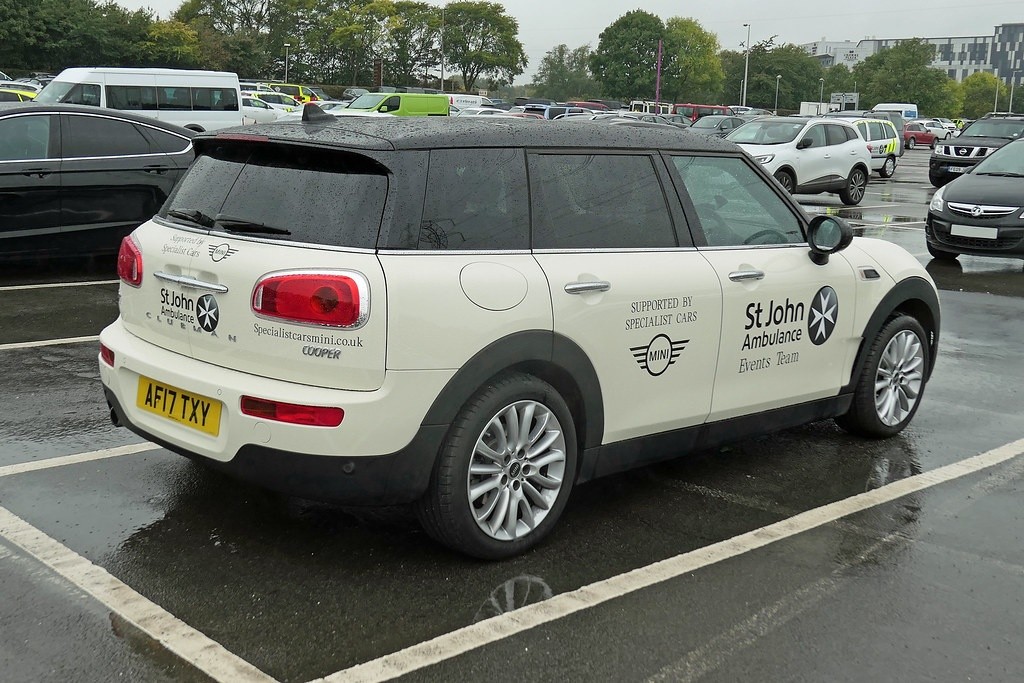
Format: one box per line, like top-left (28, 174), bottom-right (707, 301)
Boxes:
top-left (928, 111), bottom-right (1024, 190)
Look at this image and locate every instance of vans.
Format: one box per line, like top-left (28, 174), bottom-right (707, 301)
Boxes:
top-left (36, 67), bottom-right (244, 139)
top-left (872, 102), bottom-right (918, 124)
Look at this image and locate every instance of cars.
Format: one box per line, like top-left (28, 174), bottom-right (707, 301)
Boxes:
top-left (834, 117), bottom-right (904, 179)
top-left (0, 66), bottom-right (57, 104)
top-left (725, 116), bottom-right (873, 204)
top-left (240, 74), bottom-right (974, 163)
top-left (904, 122), bottom-right (940, 149)
top-left (99, 114), bottom-right (942, 564)
top-left (1, 100), bottom-right (199, 270)
top-left (924, 136), bottom-right (1024, 264)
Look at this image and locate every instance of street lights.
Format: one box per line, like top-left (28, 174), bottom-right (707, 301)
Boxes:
top-left (283, 43), bottom-right (290, 83)
top-left (1007, 69), bottom-right (1023, 110)
top-left (819, 76), bottom-right (825, 115)
top-left (741, 23), bottom-right (751, 114)
top-left (773, 74), bottom-right (783, 115)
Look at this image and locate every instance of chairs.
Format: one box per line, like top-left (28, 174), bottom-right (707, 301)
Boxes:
top-left (154, 87), bottom-right (235, 106)
top-left (767, 127), bottom-right (779, 138)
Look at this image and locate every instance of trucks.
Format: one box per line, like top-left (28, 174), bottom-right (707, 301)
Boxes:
top-left (344, 92), bottom-right (450, 118)
